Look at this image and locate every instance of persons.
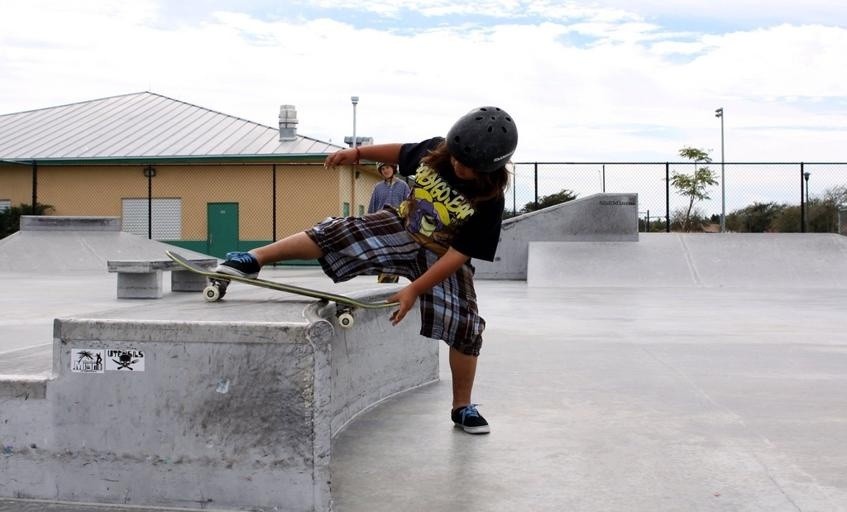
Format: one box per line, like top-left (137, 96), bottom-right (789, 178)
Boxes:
top-left (368, 159), bottom-right (410, 283)
top-left (216, 106), bottom-right (517, 434)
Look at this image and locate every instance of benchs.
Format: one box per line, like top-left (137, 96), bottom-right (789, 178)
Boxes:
top-left (106, 257), bottom-right (218, 300)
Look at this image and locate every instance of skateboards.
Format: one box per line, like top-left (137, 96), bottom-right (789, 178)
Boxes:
top-left (165, 250), bottom-right (401, 329)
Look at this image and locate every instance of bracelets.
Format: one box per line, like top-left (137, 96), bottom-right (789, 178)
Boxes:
top-left (356, 146), bottom-right (363, 164)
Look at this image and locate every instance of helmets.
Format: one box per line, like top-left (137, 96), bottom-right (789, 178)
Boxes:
top-left (447, 105), bottom-right (518, 171)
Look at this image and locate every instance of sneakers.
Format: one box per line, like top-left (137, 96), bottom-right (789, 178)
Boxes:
top-left (215, 251), bottom-right (260, 280)
top-left (451, 405), bottom-right (490, 435)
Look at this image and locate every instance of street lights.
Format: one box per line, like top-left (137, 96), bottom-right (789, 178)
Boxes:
top-left (713, 104), bottom-right (729, 233)
top-left (350, 93), bottom-right (361, 216)
top-left (802, 171), bottom-right (812, 232)
